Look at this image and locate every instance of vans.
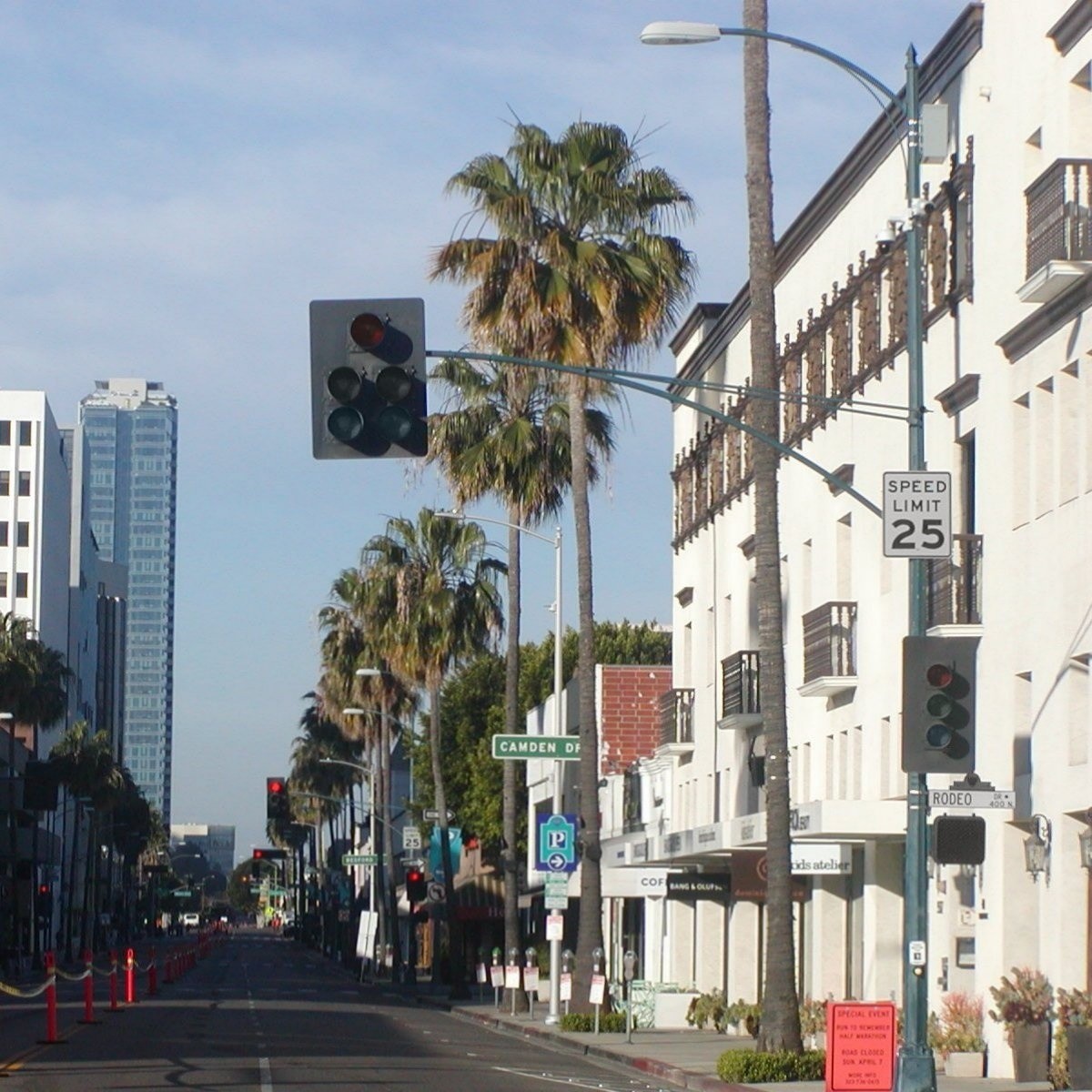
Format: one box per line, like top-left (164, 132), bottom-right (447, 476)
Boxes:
top-left (182, 913), bottom-right (200, 928)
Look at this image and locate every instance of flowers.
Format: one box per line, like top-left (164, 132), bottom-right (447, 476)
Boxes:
top-left (988, 965), bottom-right (1054, 1032)
top-left (1056, 987), bottom-right (1092, 1092)
top-left (930, 991), bottom-right (985, 1059)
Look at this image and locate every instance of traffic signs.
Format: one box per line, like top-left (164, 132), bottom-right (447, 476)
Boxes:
top-left (422, 809), bottom-right (455, 824)
top-left (536, 814), bottom-right (579, 874)
top-left (398, 858), bottom-right (425, 868)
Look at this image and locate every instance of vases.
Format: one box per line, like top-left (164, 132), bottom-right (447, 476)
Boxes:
top-left (1013, 1022), bottom-right (1051, 1082)
top-left (944, 1053), bottom-right (983, 1078)
top-left (1067, 1026), bottom-right (1091, 1091)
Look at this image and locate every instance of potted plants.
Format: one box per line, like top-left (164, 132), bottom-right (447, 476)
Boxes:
top-left (686, 988), bottom-right (829, 1053)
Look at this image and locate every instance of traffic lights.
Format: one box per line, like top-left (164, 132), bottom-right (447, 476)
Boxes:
top-left (902, 636), bottom-right (978, 775)
top-left (266, 776), bottom-right (290, 821)
top-left (308, 298), bottom-right (430, 459)
top-left (406, 871), bottom-right (426, 901)
top-left (253, 848), bottom-right (287, 860)
top-left (932, 816), bottom-right (987, 865)
top-left (37, 885), bottom-right (48, 894)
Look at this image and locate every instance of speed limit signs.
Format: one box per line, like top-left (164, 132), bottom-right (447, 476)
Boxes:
top-left (402, 826), bottom-right (421, 849)
top-left (883, 472), bottom-right (953, 561)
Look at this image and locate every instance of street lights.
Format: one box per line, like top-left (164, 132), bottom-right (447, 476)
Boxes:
top-left (343, 707), bottom-right (418, 986)
top-left (138, 841), bottom-right (187, 911)
top-left (435, 508), bottom-right (565, 1028)
top-left (320, 758), bottom-right (379, 977)
top-left (29, 796), bottom-right (92, 971)
top-left (157, 854), bottom-right (201, 941)
top-left (89, 823), bottom-right (128, 960)
top-left (637, 18), bottom-right (948, 1092)
top-left (357, 668), bottom-right (443, 997)
